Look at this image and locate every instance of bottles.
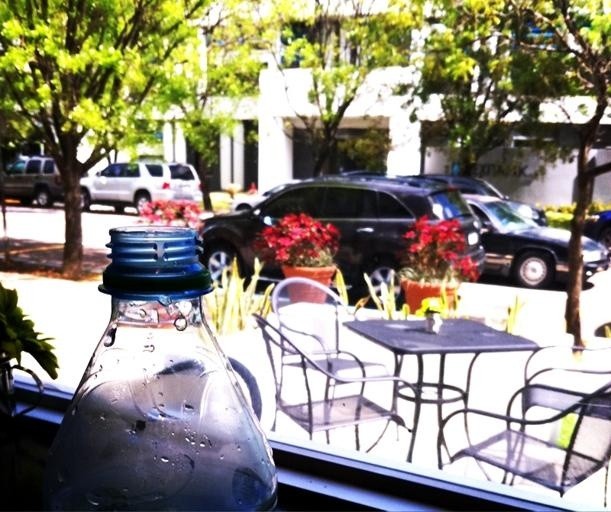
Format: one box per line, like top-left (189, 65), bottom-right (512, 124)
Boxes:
top-left (38, 224), bottom-right (278, 509)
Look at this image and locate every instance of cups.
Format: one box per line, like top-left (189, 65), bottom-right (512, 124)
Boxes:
top-left (425, 311), bottom-right (443, 334)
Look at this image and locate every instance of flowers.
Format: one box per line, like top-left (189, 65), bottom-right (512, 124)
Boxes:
top-left (134, 196), bottom-right (202, 230)
top-left (255, 212), bottom-right (347, 266)
top-left (398, 215), bottom-right (478, 283)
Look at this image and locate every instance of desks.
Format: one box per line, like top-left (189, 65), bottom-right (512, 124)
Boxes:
top-left (344, 315), bottom-right (538, 483)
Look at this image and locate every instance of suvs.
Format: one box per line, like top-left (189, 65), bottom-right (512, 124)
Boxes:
top-left (79, 161), bottom-right (203, 217)
top-left (0, 156), bottom-right (88, 208)
top-left (195, 172), bottom-right (487, 308)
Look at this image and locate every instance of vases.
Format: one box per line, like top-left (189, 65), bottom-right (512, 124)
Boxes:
top-left (283, 261), bottom-right (339, 302)
top-left (401, 279), bottom-right (460, 312)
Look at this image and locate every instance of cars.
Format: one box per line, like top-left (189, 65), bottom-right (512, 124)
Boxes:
top-left (587, 207), bottom-right (611, 248)
top-left (412, 174), bottom-right (549, 224)
top-left (232, 179), bottom-right (306, 212)
top-left (462, 192), bottom-right (609, 290)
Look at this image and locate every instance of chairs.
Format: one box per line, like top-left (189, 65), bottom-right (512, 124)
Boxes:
top-left (271, 276), bottom-right (388, 438)
top-left (254, 312), bottom-right (419, 461)
top-left (439, 366), bottom-right (611, 509)
